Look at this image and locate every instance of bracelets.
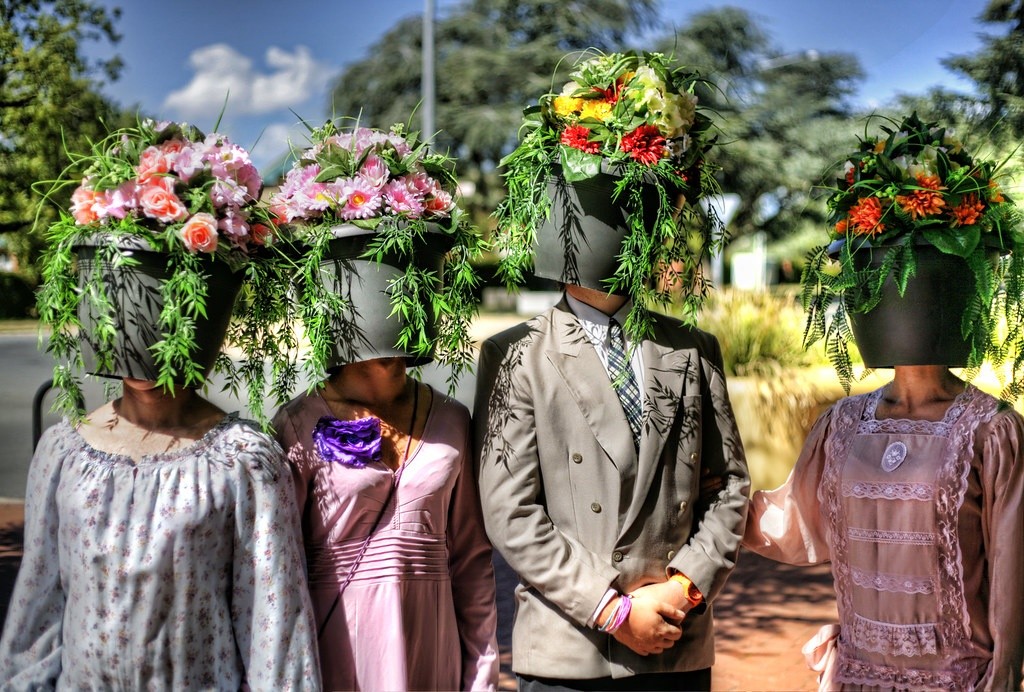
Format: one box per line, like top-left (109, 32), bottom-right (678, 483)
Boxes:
top-left (598, 594), bottom-right (633, 634)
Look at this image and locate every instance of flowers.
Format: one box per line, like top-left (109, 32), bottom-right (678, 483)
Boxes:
top-left (311, 416), bottom-right (384, 467)
top-left (266, 89), bottom-right (487, 399)
top-left (796, 108), bottom-right (1024, 405)
top-left (487, 29), bottom-right (752, 391)
top-left (23, 87), bottom-right (267, 440)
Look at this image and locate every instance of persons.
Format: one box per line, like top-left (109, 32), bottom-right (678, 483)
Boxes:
top-left (740, 364), bottom-right (1023, 692)
top-left (267, 359), bottom-right (500, 692)
top-left (472, 286), bottom-right (751, 692)
top-left (0, 378), bottom-right (323, 692)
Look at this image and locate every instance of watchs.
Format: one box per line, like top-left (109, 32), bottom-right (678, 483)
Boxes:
top-left (672, 573), bottom-right (703, 606)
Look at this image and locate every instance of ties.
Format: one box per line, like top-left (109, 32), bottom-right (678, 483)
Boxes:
top-left (608, 318), bottom-right (643, 456)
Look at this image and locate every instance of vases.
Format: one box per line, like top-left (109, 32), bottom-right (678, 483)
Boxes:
top-left (827, 237), bottom-right (973, 368)
top-left (282, 219), bottom-right (449, 368)
top-left (68, 233), bottom-right (246, 392)
top-left (528, 153), bottom-right (679, 298)
